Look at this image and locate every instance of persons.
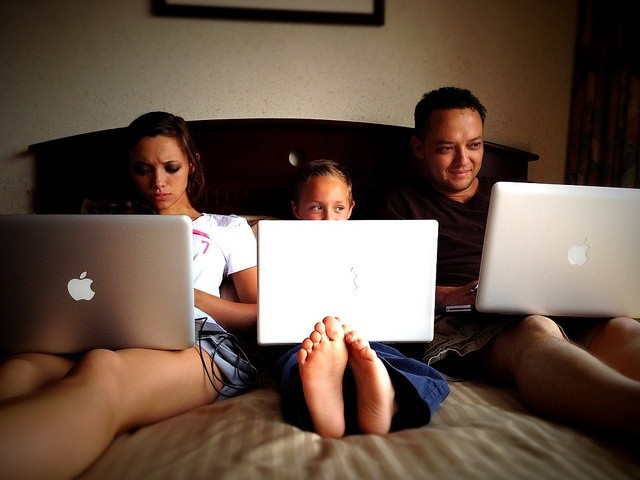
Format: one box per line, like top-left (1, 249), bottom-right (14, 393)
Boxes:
top-left (275, 158), bottom-right (450, 439)
top-left (388, 87), bottom-right (640, 436)
top-left (0, 112), bottom-right (257, 480)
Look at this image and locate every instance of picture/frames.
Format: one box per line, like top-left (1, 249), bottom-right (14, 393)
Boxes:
top-left (150, 0), bottom-right (384, 26)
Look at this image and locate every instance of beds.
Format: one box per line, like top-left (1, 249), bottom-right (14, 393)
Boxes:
top-left (28, 118), bottom-right (639, 480)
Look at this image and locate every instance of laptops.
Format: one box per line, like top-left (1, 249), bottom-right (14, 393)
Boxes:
top-left (0, 213), bottom-right (196, 350)
top-left (254, 219), bottom-right (441, 347)
top-left (474, 179), bottom-right (640, 318)
